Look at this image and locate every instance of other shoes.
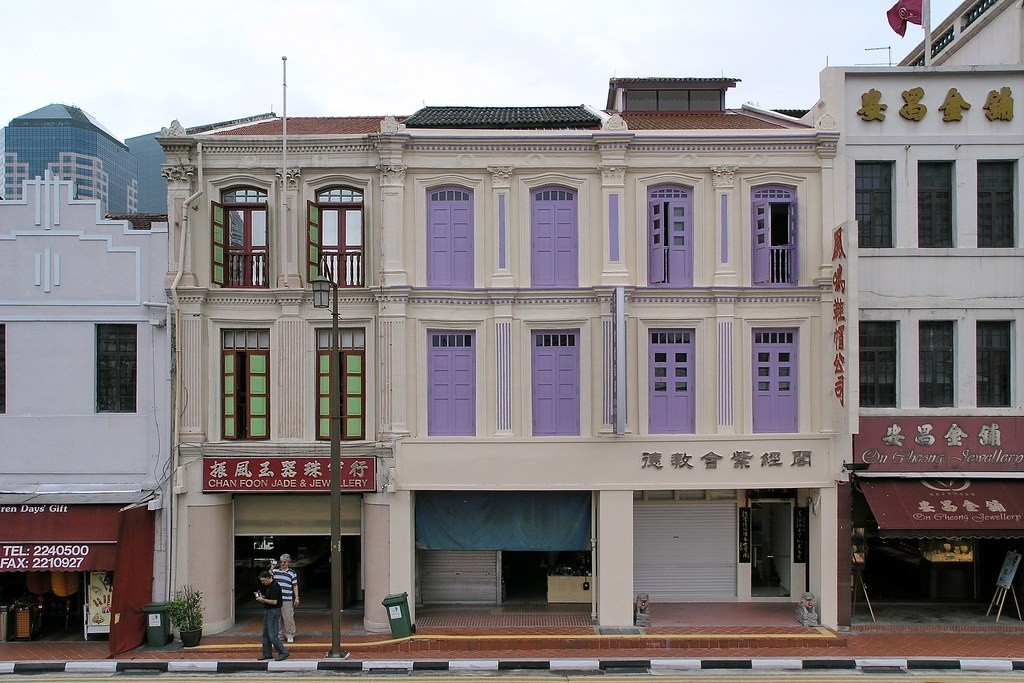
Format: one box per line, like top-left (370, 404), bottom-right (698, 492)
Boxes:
top-left (278, 634), bottom-right (284, 640)
top-left (275, 653), bottom-right (289, 661)
top-left (287, 638), bottom-right (293, 644)
top-left (257, 655), bottom-right (273, 660)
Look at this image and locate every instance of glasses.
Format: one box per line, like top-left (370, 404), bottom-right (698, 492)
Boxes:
top-left (280, 561), bottom-right (288, 564)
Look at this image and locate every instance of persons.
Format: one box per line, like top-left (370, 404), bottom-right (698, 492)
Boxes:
top-left (269, 553), bottom-right (299, 642)
top-left (255, 571), bottom-right (289, 661)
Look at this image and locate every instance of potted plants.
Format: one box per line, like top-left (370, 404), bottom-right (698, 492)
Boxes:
top-left (163, 585), bottom-right (205, 648)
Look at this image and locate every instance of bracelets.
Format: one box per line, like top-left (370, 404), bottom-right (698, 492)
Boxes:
top-left (262, 598), bottom-right (265, 603)
top-left (270, 568), bottom-right (273, 572)
top-left (294, 596), bottom-right (299, 599)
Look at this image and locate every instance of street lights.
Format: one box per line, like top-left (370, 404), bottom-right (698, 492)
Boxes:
top-left (308, 258), bottom-right (350, 660)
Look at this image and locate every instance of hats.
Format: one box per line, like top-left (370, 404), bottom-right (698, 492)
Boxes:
top-left (280, 554), bottom-right (291, 561)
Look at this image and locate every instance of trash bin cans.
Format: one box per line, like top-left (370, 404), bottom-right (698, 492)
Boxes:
top-left (142, 601), bottom-right (174, 648)
top-left (382, 591), bottom-right (416, 638)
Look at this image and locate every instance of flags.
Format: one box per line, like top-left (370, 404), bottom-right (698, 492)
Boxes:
top-left (887, 0), bottom-right (923, 38)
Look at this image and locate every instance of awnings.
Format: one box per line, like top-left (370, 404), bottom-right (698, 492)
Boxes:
top-left (0, 503), bottom-right (135, 571)
top-left (853, 472), bottom-right (1024, 538)
